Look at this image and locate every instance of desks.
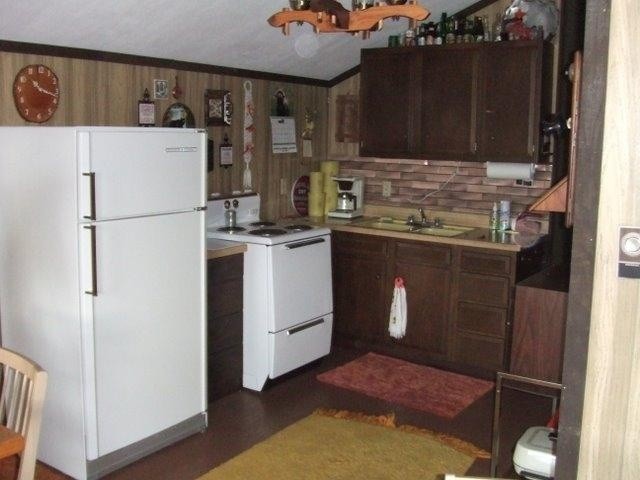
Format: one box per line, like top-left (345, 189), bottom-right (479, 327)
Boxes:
top-left (1, 424), bottom-right (25, 460)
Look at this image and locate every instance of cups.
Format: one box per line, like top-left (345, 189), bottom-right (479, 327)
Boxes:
top-left (307, 158), bottom-right (339, 218)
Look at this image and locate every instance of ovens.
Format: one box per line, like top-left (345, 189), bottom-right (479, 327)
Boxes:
top-left (243, 235), bottom-right (333, 392)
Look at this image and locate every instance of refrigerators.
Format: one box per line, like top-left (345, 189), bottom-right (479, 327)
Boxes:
top-left (0, 125), bottom-right (208, 480)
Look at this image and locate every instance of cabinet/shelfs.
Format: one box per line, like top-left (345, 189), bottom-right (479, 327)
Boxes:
top-left (360, 48), bottom-right (416, 159)
top-left (416, 39), bottom-right (555, 164)
top-left (208, 251), bottom-right (244, 397)
top-left (332, 231), bottom-right (390, 350)
top-left (394, 240), bottom-right (457, 370)
top-left (488, 369), bottom-right (562, 478)
top-left (456, 250), bottom-right (515, 381)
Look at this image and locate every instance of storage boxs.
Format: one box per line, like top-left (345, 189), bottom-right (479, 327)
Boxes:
top-left (513, 423), bottom-right (557, 479)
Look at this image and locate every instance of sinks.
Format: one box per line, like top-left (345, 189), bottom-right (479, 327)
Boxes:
top-left (344, 217), bottom-right (417, 232)
top-left (412, 222), bottom-right (475, 238)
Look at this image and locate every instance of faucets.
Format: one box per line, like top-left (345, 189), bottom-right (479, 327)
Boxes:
top-left (417, 207), bottom-right (426, 224)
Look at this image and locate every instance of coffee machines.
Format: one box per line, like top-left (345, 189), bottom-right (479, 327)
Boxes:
top-left (328, 176), bottom-right (364, 218)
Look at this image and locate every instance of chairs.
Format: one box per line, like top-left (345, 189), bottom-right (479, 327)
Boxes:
top-left (1, 343), bottom-right (50, 480)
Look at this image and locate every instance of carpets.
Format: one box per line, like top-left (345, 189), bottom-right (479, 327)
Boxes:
top-left (196, 406), bottom-right (486, 479)
top-left (318, 351), bottom-right (493, 420)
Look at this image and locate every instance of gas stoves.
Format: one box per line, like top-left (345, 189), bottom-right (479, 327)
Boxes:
top-left (209, 219), bottom-right (331, 246)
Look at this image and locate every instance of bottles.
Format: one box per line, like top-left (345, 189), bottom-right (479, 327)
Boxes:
top-left (490, 230), bottom-right (515, 245)
top-left (225, 211), bottom-right (236, 228)
top-left (390, 13), bottom-right (544, 47)
top-left (490, 200), bottom-right (518, 230)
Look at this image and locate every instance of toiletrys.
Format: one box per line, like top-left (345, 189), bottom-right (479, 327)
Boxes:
top-left (488, 230), bottom-right (501, 243)
top-left (489, 202), bottom-right (500, 230)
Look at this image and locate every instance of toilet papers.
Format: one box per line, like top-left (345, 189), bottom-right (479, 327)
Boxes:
top-left (486, 161), bottom-right (536, 180)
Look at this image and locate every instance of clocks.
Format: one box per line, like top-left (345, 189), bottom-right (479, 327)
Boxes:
top-left (14, 64), bottom-right (60, 123)
